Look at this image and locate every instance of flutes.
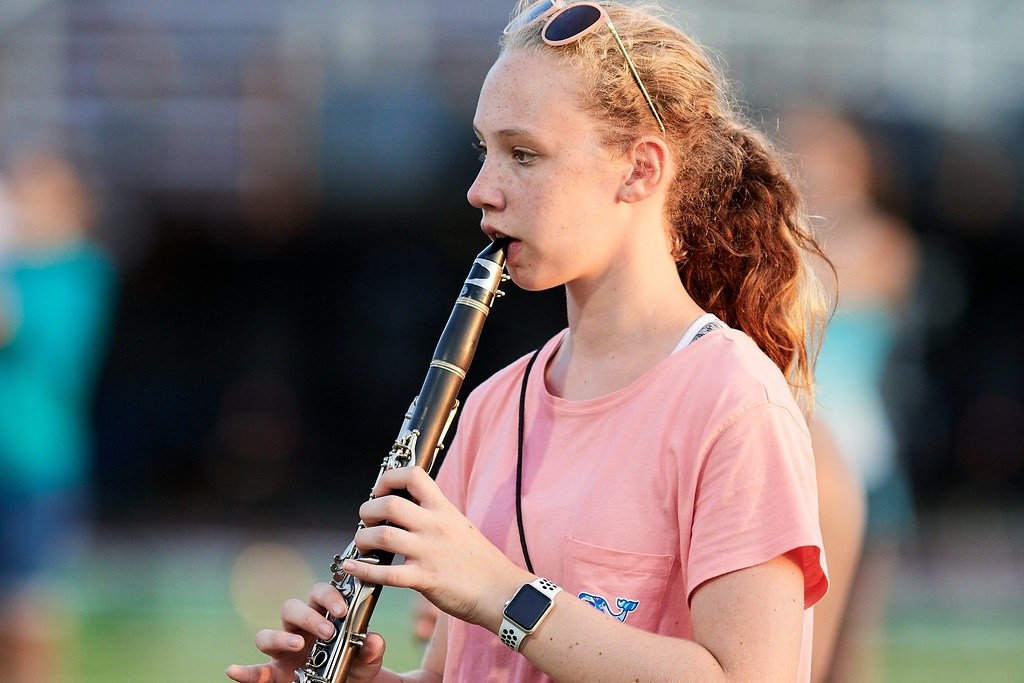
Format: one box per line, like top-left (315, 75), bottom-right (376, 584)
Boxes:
top-left (290, 233), bottom-right (516, 683)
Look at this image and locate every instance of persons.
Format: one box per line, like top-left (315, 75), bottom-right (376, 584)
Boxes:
top-left (0, 145), bottom-right (115, 683)
top-left (225, 0), bottom-right (838, 682)
top-left (776, 98), bottom-right (918, 682)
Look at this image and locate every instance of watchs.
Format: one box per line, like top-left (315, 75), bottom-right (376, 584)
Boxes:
top-left (498, 577), bottom-right (564, 653)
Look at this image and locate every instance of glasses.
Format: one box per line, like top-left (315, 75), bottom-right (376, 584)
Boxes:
top-left (503, 0), bottom-right (667, 141)
